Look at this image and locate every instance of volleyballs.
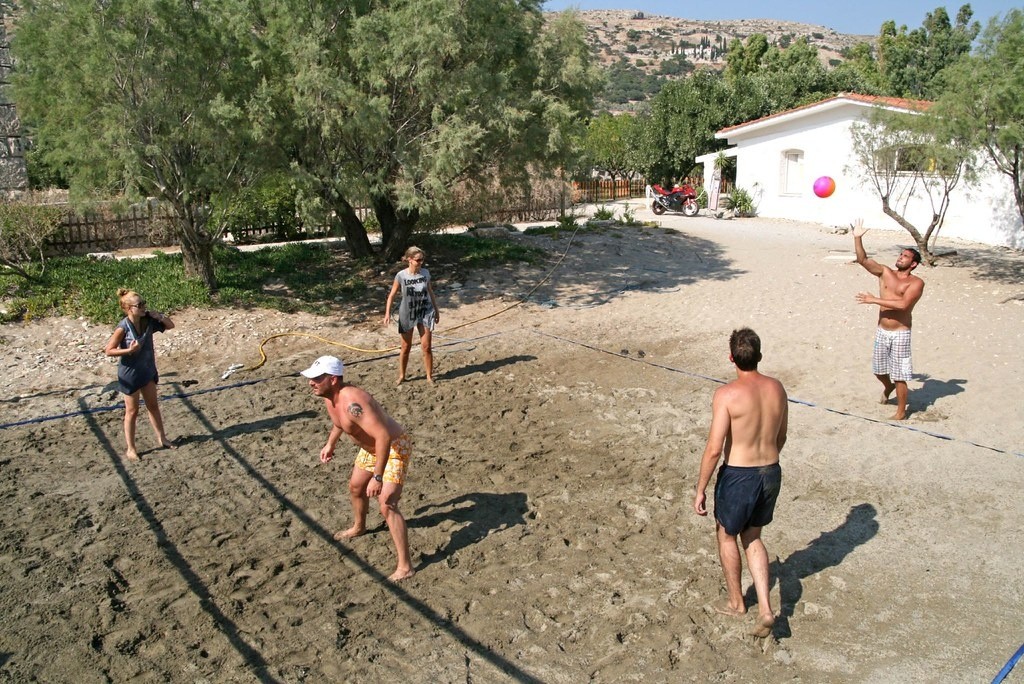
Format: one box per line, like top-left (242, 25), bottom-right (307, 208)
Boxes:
top-left (812, 176), bottom-right (836, 199)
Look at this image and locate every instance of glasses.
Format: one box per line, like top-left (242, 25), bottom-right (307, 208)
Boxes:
top-left (413, 257), bottom-right (425, 263)
top-left (129, 300), bottom-right (147, 309)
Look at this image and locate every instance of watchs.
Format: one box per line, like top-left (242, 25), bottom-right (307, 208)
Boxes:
top-left (372, 474), bottom-right (383, 482)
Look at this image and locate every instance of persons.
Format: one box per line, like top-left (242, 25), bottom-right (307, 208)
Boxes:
top-left (849, 218), bottom-right (924, 421)
top-left (106, 288), bottom-right (178, 461)
top-left (301, 355), bottom-right (415, 583)
top-left (383, 245), bottom-right (440, 388)
top-left (695, 327), bottom-right (789, 638)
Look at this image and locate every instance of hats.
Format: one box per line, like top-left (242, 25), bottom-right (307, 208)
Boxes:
top-left (300, 355), bottom-right (343, 379)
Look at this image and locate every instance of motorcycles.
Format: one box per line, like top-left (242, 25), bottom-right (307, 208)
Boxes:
top-left (652, 181), bottom-right (700, 217)
